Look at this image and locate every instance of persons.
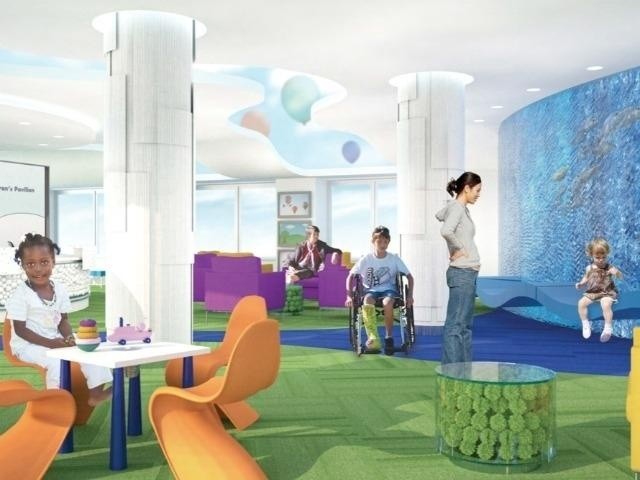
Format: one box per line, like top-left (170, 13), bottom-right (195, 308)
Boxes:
top-left (345, 225), bottom-right (415, 356)
top-left (5, 234), bottom-right (113, 426)
top-left (281, 225), bottom-right (341, 285)
top-left (575, 239), bottom-right (624, 343)
top-left (435, 171), bottom-right (481, 365)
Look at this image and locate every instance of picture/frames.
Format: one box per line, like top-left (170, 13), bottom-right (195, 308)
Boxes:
top-left (277, 191), bottom-right (312, 271)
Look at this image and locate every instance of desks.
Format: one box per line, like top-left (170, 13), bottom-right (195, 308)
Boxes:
top-left (46, 338), bottom-right (211, 470)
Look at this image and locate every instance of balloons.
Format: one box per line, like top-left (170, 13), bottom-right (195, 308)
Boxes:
top-left (280, 73), bottom-right (322, 127)
top-left (240, 110), bottom-right (270, 138)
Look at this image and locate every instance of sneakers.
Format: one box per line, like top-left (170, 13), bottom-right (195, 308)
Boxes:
top-left (384, 337), bottom-right (393, 355)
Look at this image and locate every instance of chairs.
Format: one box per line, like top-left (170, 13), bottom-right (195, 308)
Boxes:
top-left (147, 293), bottom-right (282, 480)
top-left (282, 250), bottom-right (362, 308)
top-left (193, 251), bottom-right (285, 326)
top-left (0, 308), bottom-right (105, 480)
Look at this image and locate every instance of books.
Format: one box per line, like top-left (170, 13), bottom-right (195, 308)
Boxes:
top-left (340, 140), bottom-right (360, 163)
